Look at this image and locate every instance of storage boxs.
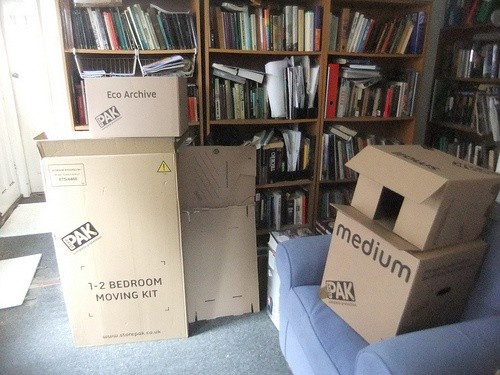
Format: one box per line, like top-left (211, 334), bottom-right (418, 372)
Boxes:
top-left (265, 227), bottom-right (325, 332)
top-left (83, 75), bottom-right (189, 140)
top-left (319, 202), bottom-right (489, 346)
top-left (330, 140), bottom-right (500, 253)
top-left (32, 129), bottom-right (189, 348)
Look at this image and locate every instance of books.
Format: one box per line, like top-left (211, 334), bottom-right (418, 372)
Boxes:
top-left (431, 0), bottom-right (500, 201)
top-left (60, 0), bottom-right (426, 238)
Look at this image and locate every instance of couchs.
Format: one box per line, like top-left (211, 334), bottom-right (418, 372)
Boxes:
top-left (276, 200), bottom-right (500, 375)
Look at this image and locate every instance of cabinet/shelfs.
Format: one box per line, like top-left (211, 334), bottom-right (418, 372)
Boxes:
top-left (54, 0), bottom-right (432, 263)
top-left (424, 0), bottom-right (500, 220)
